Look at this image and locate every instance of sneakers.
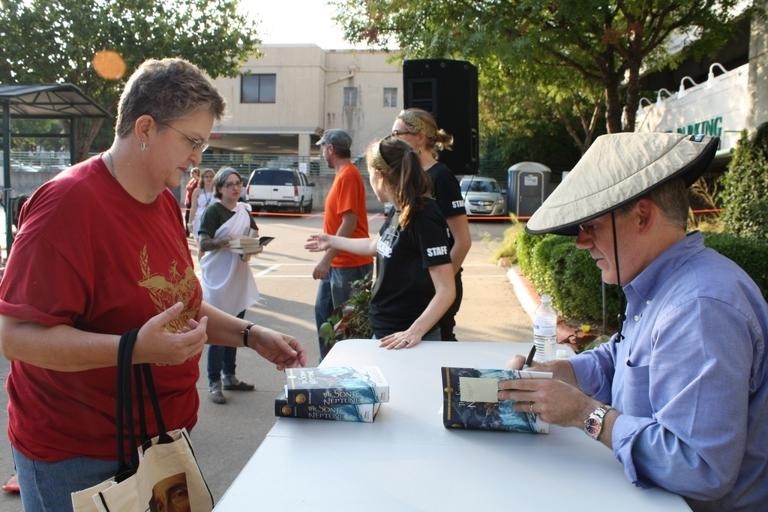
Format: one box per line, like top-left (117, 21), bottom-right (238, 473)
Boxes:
top-left (224, 381), bottom-right (254, 390)
top-left (208, 391), bottom-right (225, 403)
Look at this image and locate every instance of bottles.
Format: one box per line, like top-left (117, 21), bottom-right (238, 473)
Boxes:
top-left (532, 293), bottom-right (557, 362)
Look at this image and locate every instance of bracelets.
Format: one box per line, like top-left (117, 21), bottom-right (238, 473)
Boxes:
top-left (243, 323), bottom-right (255, 347)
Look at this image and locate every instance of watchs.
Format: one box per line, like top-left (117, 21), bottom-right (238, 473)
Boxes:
top-left (583, 404), bottom-right (616, 441)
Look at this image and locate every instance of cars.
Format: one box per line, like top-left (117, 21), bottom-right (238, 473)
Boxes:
top-left (454, 175), bottom-right (505, 217)
top-left (236, 176), bottom-right (248, 202)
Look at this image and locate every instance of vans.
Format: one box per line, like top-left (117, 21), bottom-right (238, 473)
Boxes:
top-left (246, 167), bottom-right (315, 214)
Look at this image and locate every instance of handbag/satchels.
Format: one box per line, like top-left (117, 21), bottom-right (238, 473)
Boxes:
top-left (71, 427), bottom-right (214, 512)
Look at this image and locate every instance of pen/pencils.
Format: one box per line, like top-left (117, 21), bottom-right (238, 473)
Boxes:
top-left (522, 345), bottom-right (536, 370)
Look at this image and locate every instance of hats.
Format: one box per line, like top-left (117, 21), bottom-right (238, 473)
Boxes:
top-left (315, 129), bottom-right (352, 148)
top-left (525, 132), bottom-right (719, 235)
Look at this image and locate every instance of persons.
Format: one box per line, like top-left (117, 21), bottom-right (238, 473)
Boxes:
top-left (1, 57), bottom-right (308, 512)
top-left (304, 135), bottom-right (458, 350)
top-left (197, 166), bottom-right (258, 404)
top-left (312, 129), bottom-right (373, 363)
top-left (497, 131), bottom-right (768, 512)
top-left (185, 168), bottom-right (201, 237)
top-left (392, 107), bottom-right (472, 342)
top-left (187, 168), bottom-right (216, 247)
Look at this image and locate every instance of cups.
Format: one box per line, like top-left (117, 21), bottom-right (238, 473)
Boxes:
top-left (581, 324), bottom-right (591, 333)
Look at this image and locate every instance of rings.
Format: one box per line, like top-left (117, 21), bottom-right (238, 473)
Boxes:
top-left (528, 402), bottom-right (533, 412)
top-left (402, 339), bottom-right (409, 344)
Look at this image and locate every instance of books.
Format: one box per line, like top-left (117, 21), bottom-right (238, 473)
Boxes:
top-left (274, 394), bottom-right (381, 423)
top-left (283, 365), bottom-right (389, 408)
top-left (441, 367), bottom-right (553, 435)
top-left (227, 236), bottom-right (274, 254)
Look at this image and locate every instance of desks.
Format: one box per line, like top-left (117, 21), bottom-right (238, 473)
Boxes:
top-left (210, 338), bottom-right (696, 512)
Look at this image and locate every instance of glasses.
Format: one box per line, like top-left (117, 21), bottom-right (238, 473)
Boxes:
top-left (223, 181), bottom-right (243, 188)
top-left (161, 123), bottom-right (210, 155)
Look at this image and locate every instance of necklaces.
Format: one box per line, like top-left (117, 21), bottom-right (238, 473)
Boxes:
top-left (203, 187), bottom-right (213, 205)
